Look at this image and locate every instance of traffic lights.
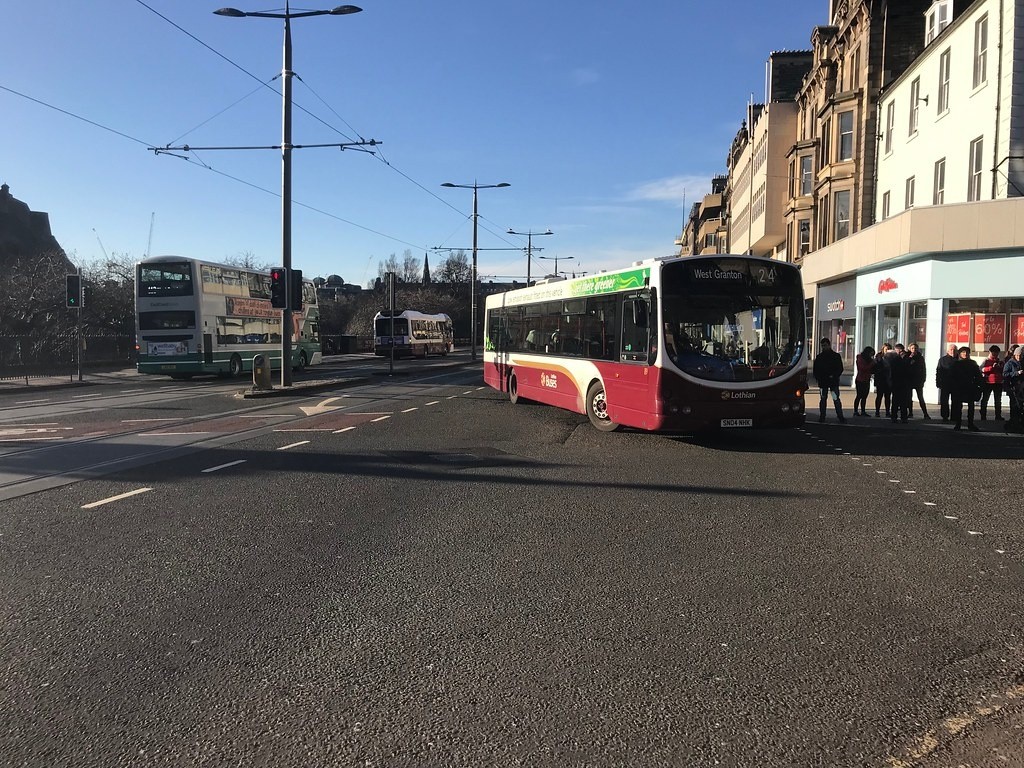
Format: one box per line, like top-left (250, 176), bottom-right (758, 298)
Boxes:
top-left (269, 268), bottom-right (288, 307)
top-left (67, 274), bottom-right (81, 306)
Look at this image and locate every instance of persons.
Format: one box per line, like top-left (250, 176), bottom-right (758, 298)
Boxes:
top-left (526, 329), bottom-right (536, 351)
top-left (812, 338), bottom-right (848, 424)
top-left (853, 346), bottom-right (877, 417)
top-left (874, 343), bottom-right (932, 424)
top-left (936, 344), bottom-right (1024, 431)
top-left (979, 345), bottom-right (1006, 420)
top-left (681, 333), bottom-right (698, 353)
top-left (779, 342), bottom-right (795, 365)
top-left (551, 329), bottom-right (560, 343)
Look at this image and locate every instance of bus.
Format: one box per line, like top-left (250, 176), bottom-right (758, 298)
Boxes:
top-left (374, 309), bottom-right (453, 359)
top-left (132, 256), bottom-right (322, 378)
top-left (483, 254), bottom-right (809, 432)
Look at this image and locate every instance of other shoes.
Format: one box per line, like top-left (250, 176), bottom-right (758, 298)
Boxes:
top-left (886, 414), bottom-right (891, 418)
top-left (981, 416), bottom-right (986, 420)
top-left (819, 416), bottom-right (825, 422)
top-left (853, 412), bottom-right (860, 416)
top-left (950, 418), bottom-right (962, 422)
top-left (924, 415), bottom-right (931, 419)
top-left (876, 413), bottom-right (880, 417)
top-left (943, 417), bottom-right (948, 421)
top-left (902, 418), bottom-right (908, 423)
top-left (995, 416), bottom-right (1004, 420)
top-left (968, 424), bottom-right (979, 431)
top-left (839, 417), bottom-right (848, 424)
top-left (954, 425), bottom-right (960, 431)
top-left (891, 418), bottom-right (901, 423)
top-left (907, 414), bottom-right (913, 418)
top-left (861, 412), bottom-right (871, 417)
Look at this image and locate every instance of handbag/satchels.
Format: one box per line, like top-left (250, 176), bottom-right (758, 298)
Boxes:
top-left (970, 383), bottom-right (981, 401)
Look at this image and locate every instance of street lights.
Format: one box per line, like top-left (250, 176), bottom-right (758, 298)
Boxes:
top-left (441, 181), bottom-right (512, 362)
top-left (559, 271), bottom-right (588, 278)
top-left (539, 256), bottom-right (574, 277)
top-left (212, 6), bottom-right (363, 384)
top-left (506, 229), bottom-right (554, 286)
